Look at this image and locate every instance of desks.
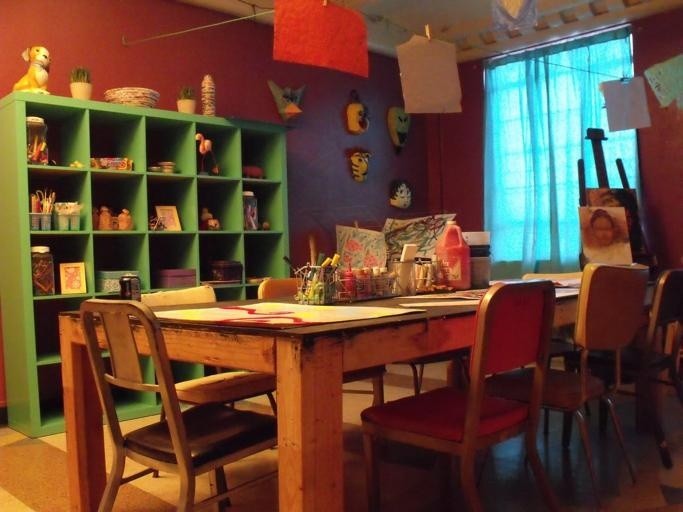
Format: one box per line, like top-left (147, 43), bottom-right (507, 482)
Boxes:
top-left (57, 278), bottom-right (656, 512)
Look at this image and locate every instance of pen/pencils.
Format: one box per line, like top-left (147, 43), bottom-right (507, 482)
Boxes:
top-left (283, 256), bottom-right (300, 276)
top-left (305, 235), bottom-right (340, 305)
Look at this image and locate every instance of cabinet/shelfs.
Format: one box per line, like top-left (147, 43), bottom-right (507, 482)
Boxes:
top-left (0, 90), bottom-right (290, 440)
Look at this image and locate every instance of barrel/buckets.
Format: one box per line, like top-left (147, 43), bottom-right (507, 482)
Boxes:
top-left (435, 219), bottom-right (472, 291)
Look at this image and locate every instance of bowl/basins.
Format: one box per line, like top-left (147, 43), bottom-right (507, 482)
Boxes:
top-left (149, 166), bottom-right (162, 171)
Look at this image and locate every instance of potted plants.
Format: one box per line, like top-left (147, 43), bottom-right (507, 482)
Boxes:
top-left (69, 65), bottom-right (92, 100)
top-left (176, 86), bottom-right (196, 113)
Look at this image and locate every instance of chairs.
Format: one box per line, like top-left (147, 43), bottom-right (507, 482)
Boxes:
top-left (141, 284), bottom-right (278, 442)
top-left (360, 279), bottom-right (561, 512)
top-left (483, 262), bottom-right (651, 510)
top-left (562, 268), bottom-right (683, 470)
top-left (79, 298), bottom-right (277, 511)
top-left (257, 277), bottom-right (385, 408)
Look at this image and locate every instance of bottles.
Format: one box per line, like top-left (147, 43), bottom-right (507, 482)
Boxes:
top-left (31, 246), bottom-right (54, 296)
top-left (373, 268), bottom-right (382, 296)
top-left (380, 267), bottom-right (392, 295)
top-left (355, 269), bottom-right (366, 298)
top-left (25, 117), bottom-right (48, 165)
top-left (243, 191), bottom-right (258, 231)
top-left (363, 268), bottom-right (373, 295)
top-left (344, 263), bottom-right (356, 298)
